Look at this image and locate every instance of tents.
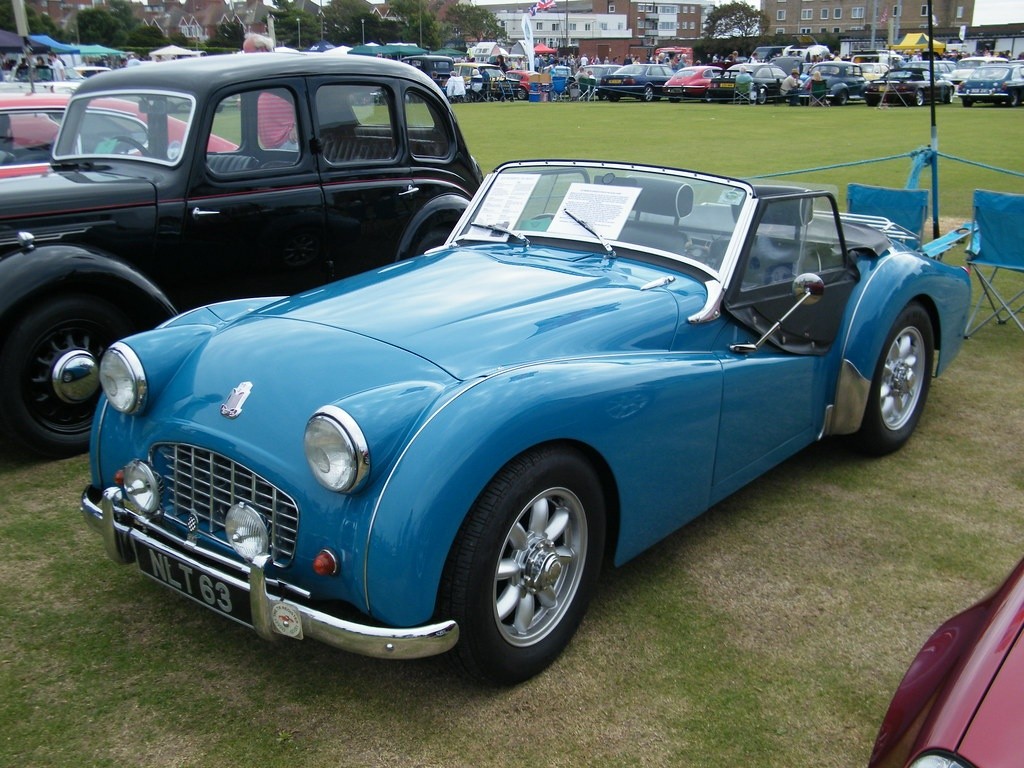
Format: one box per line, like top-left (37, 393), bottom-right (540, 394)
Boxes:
top-left (533, 42), bottom-right (557, 53)
top-left (885, 33), bottom-right (945, 53)
top-left (72, 44), bottom-right (125, 58)
top-left (148, 45), bottom-right (197, 61)
top-left (25, 35), bottom-right (80, 66)
top-left (302, 39), bottom-right (466, 59)
top-left (0, 29), bottom-right (51, 54)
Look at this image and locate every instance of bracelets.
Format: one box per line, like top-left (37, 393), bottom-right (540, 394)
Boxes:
top-left (236, 98), bottom-right (241, 102)
top-left (293, 123), bottom-right (296, 125)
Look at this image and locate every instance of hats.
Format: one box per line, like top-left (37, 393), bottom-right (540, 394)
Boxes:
top-left (792, 69), bottom-right (799, 74)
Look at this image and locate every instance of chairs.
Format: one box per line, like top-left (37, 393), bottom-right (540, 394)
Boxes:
top-left (596, 175), bottom-right (823, 285)
top-left (372, 77), bottom-right (492, 103)
top-left (207, 154), bottom-right (296, 171)
top-left (734, 81), bottom-right (758, 105)
top-left (844, 182), bottom-right (1024, 338)
top-left (808, 79), bottom-right (831, 108)
top-left (551, 75), bottom-right (597, 102)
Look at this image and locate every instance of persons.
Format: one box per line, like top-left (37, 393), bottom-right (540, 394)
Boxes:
top-left (385, 54), bottom-right (392, 59)
top-left (432, 67), bottom-right (490, 103)
top-left (35, 55), bottom-right (51, 81)
top-left (377, 52), bottom-right (383, 57)
top-left (83, 54), bottom-right (143, 78)
top-left (470, 57), bottom-right (475, 62)
top-left (574, 67), bottom-right (596, 82)
top-left (534, 53), bottom-right (642, 73)
top-left (496, 55), bottom-right (525, 74)
top-left (649, 48), bottom-right (1024, 106)
top-left (155, 55), bottom-right (161, 61)
top-left (236, 31), bottom-right (298, 151)
top-left (9, 58), bottom-right (29, 82)
top-left (200, 52), bottom-right (207, 56)
top-left (46, 53), bottom-right (67, 81)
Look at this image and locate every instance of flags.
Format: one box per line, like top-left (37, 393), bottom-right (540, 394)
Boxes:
top-left (881, 5), bottom-right (888, 26)
top-left (529, 0), bottom-right (556, 16)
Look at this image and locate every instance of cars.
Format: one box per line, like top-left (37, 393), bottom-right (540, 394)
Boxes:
top-left (949, 56), bottom-right (1008, 87)
top-left (381, 46), bottom-right (961, 105)
top-left (955, 63), bottom-right (1024, 107)
top-left (0, 93), bottom-right (244, 178)
top-left (73, 66), bottom-right (111, 81)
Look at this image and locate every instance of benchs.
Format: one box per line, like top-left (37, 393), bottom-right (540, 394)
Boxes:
top-left (320, 131), bottom-right (443, 162)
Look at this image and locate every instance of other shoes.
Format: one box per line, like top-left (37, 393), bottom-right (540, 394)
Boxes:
top-left (790, 103), bottom-right (800, 106)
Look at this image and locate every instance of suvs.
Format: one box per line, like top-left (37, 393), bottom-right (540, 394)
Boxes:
top-left (0, 51), bottom-right (487, 467)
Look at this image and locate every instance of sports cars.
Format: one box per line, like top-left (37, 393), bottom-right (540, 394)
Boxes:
top-left (76, 151), bottom-right (972, 684)
